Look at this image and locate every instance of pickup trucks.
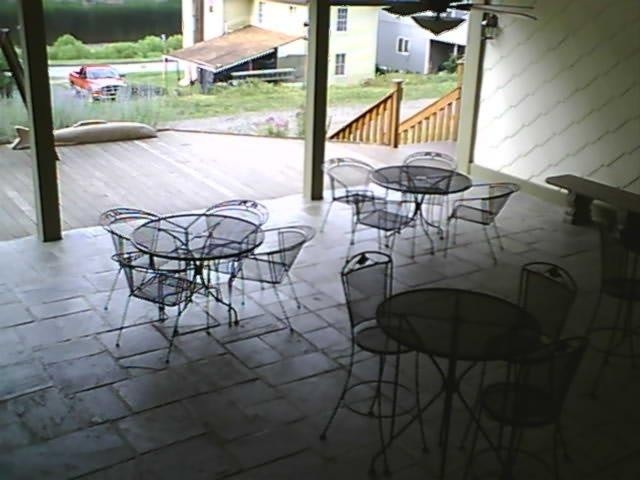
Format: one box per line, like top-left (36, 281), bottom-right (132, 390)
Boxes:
top-left (69, 63), bottom-right (129, 103)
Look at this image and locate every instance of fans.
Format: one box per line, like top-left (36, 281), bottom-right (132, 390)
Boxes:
top-left (383, 1), bottom-right (537, 35)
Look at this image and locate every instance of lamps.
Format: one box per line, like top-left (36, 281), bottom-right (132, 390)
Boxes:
top-left (411, 12), bottom-right (466, 35)
top-left (481, 13), bottom-right (498, 39)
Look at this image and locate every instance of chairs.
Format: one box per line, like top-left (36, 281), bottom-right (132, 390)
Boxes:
top-left (321, 148), bottom-right (520, 268)
top-left (100, 199), bottom-right (317, 333)
top-left (458, 220), bottom-right (639, 480)
top-left (110, 251), bottom-right (211, 364)
top-left (318, 251), bottom-right (430, 475)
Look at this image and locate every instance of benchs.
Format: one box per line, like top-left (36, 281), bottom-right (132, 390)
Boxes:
top-left (545, 174), bottom-right (639, 233)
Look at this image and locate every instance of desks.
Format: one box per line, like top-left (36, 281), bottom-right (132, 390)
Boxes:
top-left (369, 287), bottom-right (539, 480)
